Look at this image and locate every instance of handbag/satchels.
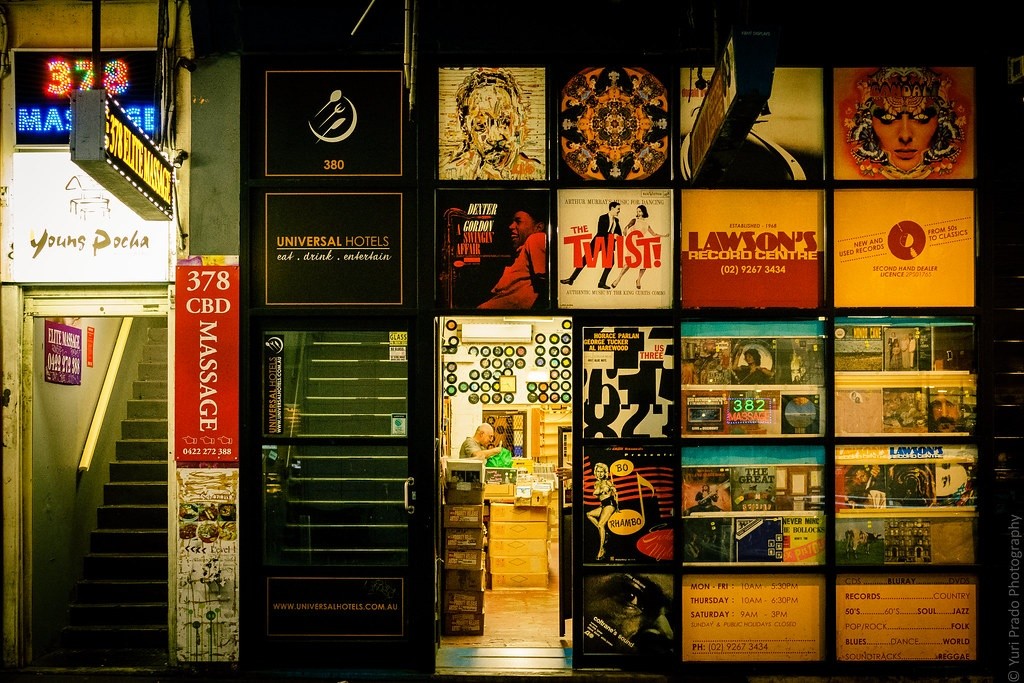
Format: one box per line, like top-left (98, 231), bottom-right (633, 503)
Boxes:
top-left (485, 447), bottom-right (513, 468)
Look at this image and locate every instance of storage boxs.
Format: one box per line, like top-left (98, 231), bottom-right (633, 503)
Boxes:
top-left (445, 458), bottom-right (558, 636)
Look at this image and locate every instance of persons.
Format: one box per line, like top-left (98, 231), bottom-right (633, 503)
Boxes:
top-left (460, 423), bottom-right (501, 458)
top-left (845, 464), bottom-right (885, 509)
top-left (560, 202), bottom-right (622, 290)
top-left (586, 463), bottom-right (619, 560)
top-left (733, 348), bottom-right (772, 376)
top-left (929, 387), bottom-right (972, 433)
top-left (611, 205), bottom-right (670, 289)
top-left (583, 573), bottom-right (672, 654)
top-left (475, 211), bottom-right (546, 310)
top-left (936, 463), bottom-right (967, 497)
top-left (888, 332), bottom-right (916, 371)
top-left (696, 485), bottom-right (718, 505)
